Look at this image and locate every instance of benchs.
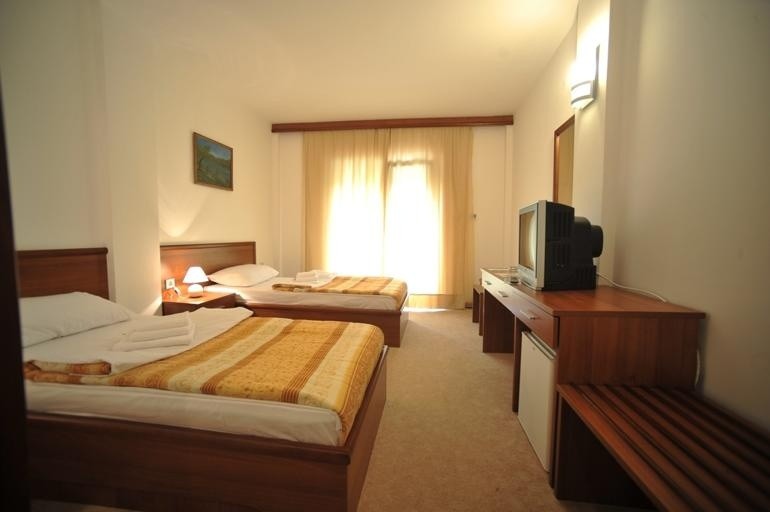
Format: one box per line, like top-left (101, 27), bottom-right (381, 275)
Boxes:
top-left (553, 382), bottom-right (770, 511)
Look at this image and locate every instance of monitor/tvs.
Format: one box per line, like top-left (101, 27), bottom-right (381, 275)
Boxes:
top-left (517, 200), bottom-right (603, 291)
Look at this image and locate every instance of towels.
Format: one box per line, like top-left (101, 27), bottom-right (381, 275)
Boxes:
top-left (297, 269), bottom-right (332, 282)
top-left (123, 312), bottom-right (190, 350)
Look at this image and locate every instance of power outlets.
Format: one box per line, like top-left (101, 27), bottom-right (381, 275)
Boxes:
top-left (165, 278), bottom-right (176, 289)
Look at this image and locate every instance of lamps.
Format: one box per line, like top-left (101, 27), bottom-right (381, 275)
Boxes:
top-left (182, 266), bottom-right (208, 297)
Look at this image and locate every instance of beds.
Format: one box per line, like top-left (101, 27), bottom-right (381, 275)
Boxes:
top-left (160, 242), bottom-right (409, 348)
top-left (14, 247), bottom-right (388, 512)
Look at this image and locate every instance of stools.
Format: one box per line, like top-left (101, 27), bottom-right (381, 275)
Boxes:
top-left (471, 271), bottom-right (483, 336)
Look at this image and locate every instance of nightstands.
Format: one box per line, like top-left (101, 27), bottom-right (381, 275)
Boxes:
top-left (162, 291), bottom-right (236, 316)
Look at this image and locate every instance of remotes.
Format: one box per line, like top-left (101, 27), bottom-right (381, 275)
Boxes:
top-left (511, 276), bottom-right (518, 283)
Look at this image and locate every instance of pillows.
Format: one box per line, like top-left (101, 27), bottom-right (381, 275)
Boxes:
top-left (18, 291), bottom-right (132, 348)
top-left (208, 263), bottom-right (280, 287)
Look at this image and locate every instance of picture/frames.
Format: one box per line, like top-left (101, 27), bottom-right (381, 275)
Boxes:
top-left (192, 132), bottom-right (234, 190)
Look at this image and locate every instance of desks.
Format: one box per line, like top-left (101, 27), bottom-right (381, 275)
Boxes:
top-left (479, 268), bottom-right (706, 488)
